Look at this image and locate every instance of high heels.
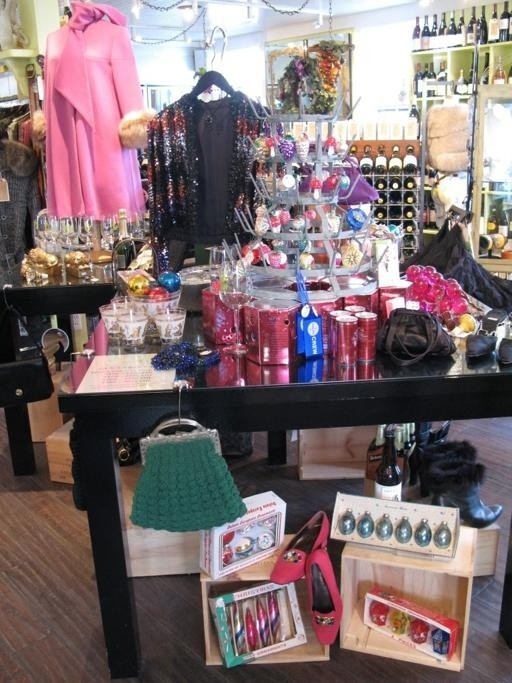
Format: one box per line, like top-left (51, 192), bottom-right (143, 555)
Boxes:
top-left (465, 308), bottom-right (508, 358)
top-left (430, 460), bottom-right (503, 529)
top-left (498, 310), bottom-right (512, 366)
top-left (420, 438), bottom-right (477, 500)
top-left (304, 549), bottom-right (342, 646)
top-left (268, 509), bottom-right (331, 585)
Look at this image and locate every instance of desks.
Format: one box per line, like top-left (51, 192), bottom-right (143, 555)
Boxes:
top-left (0, 259), bottom-right (120, 477)
top-left (57, 285), bottom-right (512, 683)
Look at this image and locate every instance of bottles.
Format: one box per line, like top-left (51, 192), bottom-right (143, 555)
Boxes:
top-left (359, 145), bottom-right (373, 174)
top-left (403, 221), bottom-right (416, 233)
top-left (480, 53), bottom-right (489, 84)
top-left (374, 177), bottom-right (387, 190)
top-left (454, 68), bottom-right (467, 94)
top-left (375, 146), bottom-right (387, 173)
top-left (116, 209), bottom-right (137, 271)
top-left (335, 315), bottom-right (358, 368)
top-left (355, 312), bottom-right (378, 363)
top-left (429, 14), bottom-right (438, 49)
top-left (410, 422), bottom-right (415, 445)
top-left (421, 15), bottom-right (430, 50)
top-left (413, 64), bottom-right (422, 97)
top-left (404, 424), bottom-right (411, 449)
top-left (422, 63), bottom-right (429, 80)
top-left (498, 207), bottom-right (508, 238)
top-left (369, 425), bottom-right (385, 451)
top-left (499, 1), bottom-right (510, 42)
top-left (404, 176), bottom-right (417, 190)
top-left (404, 206), bottom-right (416, 220)
top-left (428, 63), bottom-right (435, 96)
top-left (446, 11), bottom-right (456, 47)
top-left (467, 52), bottom-right (473, 94)
top-left (412, 16), bottom-right (421, 52)
top-left (438, 12), bottom-right (447, 49)
top-left (349, 146), bottom-right (358, 170)
top-left (488, 208), bottom-right (497, 234)
top-left (426, 170), bottom-right (437, 229)
top-left (403, 146), bottom-right (417, 174)
top-left (373, 431), bottom-right (402, 501)
top-left (404, 191), bottom-right (416, 204)
top-left (388, 145), bottom-right (403, 175)
top-left (374, 192), bottom-right (386, 205)
top-left (466, 6), bottom-right (476, 46)
top-left (344, 305), bottom-right (365, 316)
top-left (487, 2), bottom-right (500, 43)
top-left (389, 176), bottom-right (402, 190)
top-left (508, 11), bottom-right (512, 41)
top-left (492, 56), bottom-right (507, 86)
top-left (456, 9), bottom-right (465, 47)
top-left (395, 427), bottom-right (404, 457)
top-left (435, 62), bottom-right (447, 97)
top-left (423, 168), bottom-right (428, 230)
top-left (374, 206), bottom-right (387, 219)
top-left (328, 310), bottom-right (352, 359)
top-left (477, 5), bottom-right (488, 45)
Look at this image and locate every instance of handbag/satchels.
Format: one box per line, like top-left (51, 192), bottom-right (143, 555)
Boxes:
top-left (0, 302), bottom-right (53, 410)
top-left (375, 306), bottom-right (457, 368)
top-left (298, 155), bottom-right (380, 207)
top-left (129, 415), bottom-right (247, 532)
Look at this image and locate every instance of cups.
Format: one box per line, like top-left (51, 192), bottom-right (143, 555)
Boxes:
top-left (111, 295), bottom-right (130, 310)
top-left (100, 304), bottom-right (123, 338)
top-left (117, 310), bottom-right (147, 348)
top-left (58, 217), bottom-right (78, 257)
top-left (79, 216), bottom-right (99, 276)
top-left (38, 217), bottom-right (57, 252)
top-left (209, 246), bottom-right (237, 297)
top-left (219, 271), bottom-right (251, 353)
top-left (100, 215), bottom-right (119, 253)
top-left (151, 308), bottom-right (185, 344)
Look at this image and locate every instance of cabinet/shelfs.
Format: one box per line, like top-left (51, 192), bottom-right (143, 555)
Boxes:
top-left (346, 138), bottom-right (423, 263)
top-left (409, 40), bottom-right (512, 105)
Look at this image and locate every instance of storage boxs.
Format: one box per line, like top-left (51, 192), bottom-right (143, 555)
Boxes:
top-left (199, 533), bottom-right (330, 667)
top-left (339, 525), bottom-right (478, 672)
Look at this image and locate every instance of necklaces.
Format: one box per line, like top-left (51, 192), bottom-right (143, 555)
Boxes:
top-left (193, 91), bottom-right (231, 129)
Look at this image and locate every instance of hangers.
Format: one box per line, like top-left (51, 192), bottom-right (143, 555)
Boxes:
top-left (188, 26), bottom-right (236, 100)
